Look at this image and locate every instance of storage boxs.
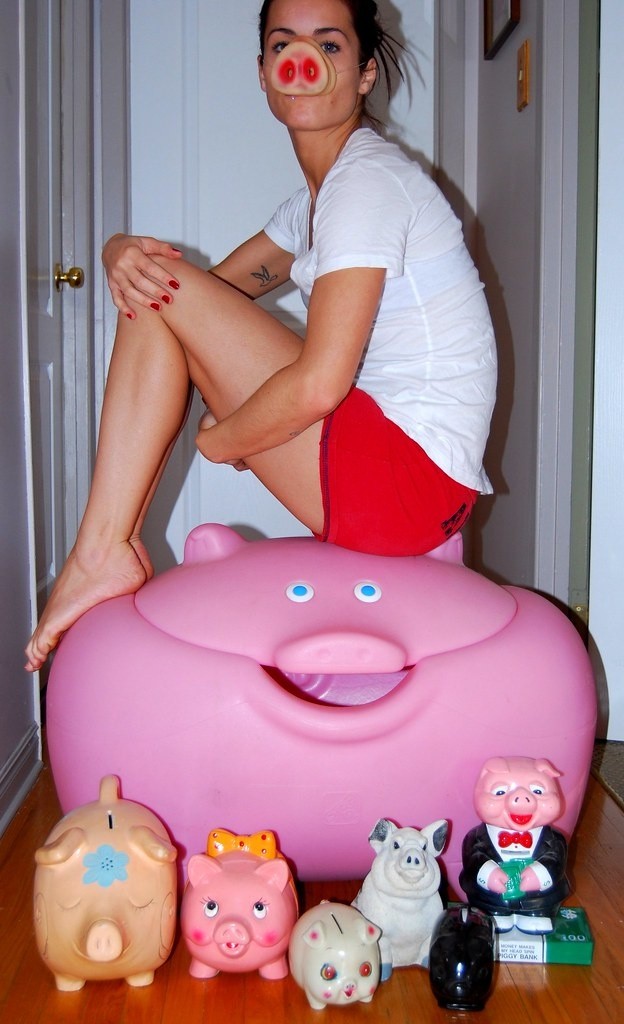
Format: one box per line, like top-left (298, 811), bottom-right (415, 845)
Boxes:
top-left (447, 900), bottom-right (594, 967)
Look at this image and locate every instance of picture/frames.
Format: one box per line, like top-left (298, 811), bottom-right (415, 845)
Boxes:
top-left (484, 0), bottom-right (521, 61)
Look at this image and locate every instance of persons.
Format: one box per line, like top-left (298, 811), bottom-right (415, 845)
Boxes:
top-left (22, 1), bottom-right (503, 674)
